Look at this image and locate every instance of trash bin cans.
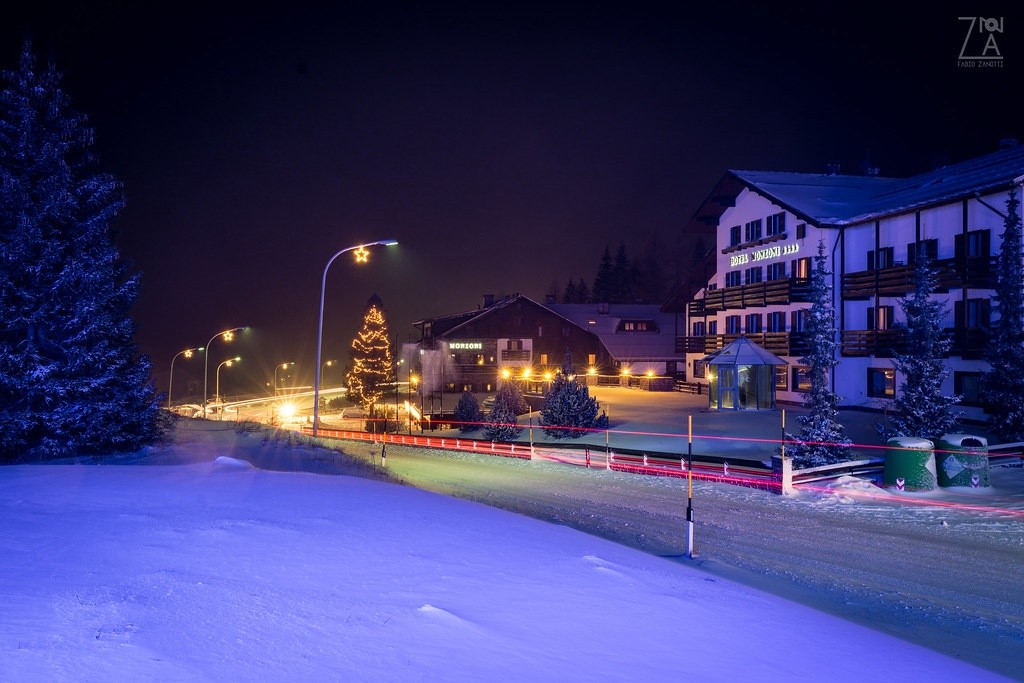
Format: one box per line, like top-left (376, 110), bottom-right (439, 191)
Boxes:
top-left (882, 437), bottom-right (937, 492)
top-left (935, 433), bottom-right (991, 489)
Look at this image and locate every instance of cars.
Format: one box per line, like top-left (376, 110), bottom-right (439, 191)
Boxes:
top-left (339, 408), bottom-right (370, 419)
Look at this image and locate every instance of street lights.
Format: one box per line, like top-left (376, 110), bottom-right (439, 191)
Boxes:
top-left (168, 347), bottom-right (205, 412)
top-left (274, 362), bottom-right (296, 396)
top-left (204, 327), bottom-right (250, 419)
top-left (313, 238), bottom-right (399, 441)
top-left (321, 360), bottom-right (338, 389)
top-left (217, 357), bottom-right (240, 418)
